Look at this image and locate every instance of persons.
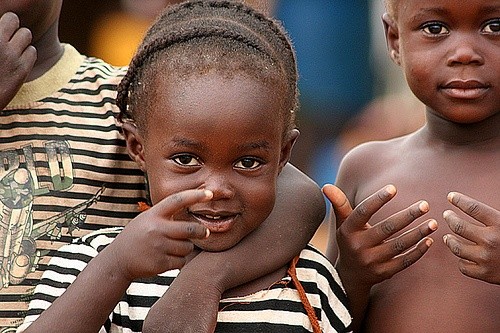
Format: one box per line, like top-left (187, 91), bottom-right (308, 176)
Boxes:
top-left (12, 0), bottom-right (355, 333)
top-left (321, 0), bottom-right (500, 332)
top-left (1, 0), bottom-right (327, 333)
